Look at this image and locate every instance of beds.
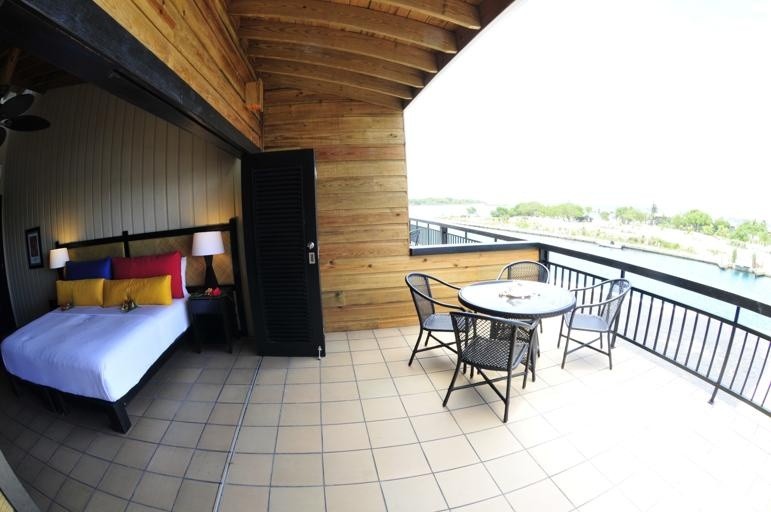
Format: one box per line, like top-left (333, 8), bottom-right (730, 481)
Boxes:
top-left (0, 217), bottom-right (243, 435)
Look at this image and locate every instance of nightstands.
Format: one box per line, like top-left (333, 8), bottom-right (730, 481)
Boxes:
top-left (187, 286), bottom-right (239, 353)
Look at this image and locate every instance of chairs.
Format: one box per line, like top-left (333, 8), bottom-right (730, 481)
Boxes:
top-left (496, 261), bottom-right (549, 283)
top-left (558, 279), bottom-right (630, 368)
top-left (444, 311), bottom-right (538, 423)
top-left (405, 273), bottom-right (473, 365)
top-left (410, 228), bottom-right (421, 246)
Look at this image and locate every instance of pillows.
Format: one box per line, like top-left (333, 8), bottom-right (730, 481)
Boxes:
top-left (67, 256), bottom-right (113, 280)
top-left (111, 249), bottom-right (184, 298)
top-left (103, 274), bottom-right (172, 308)
top-left (56, 278), bottom-right (104, 306)
top-left (180, 256), bottom-right (188, 296)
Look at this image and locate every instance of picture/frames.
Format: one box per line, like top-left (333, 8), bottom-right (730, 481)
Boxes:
top-left (25, 227), bottom-right (44, 269)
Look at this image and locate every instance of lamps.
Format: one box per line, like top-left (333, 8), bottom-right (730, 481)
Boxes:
top-left (192, 231), bottom-right (226, 287)
top-left (48, 249), bottom-right (69, 280)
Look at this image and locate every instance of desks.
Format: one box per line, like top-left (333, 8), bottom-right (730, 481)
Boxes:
top-left (457, 281), bottom-right (578, 359)
